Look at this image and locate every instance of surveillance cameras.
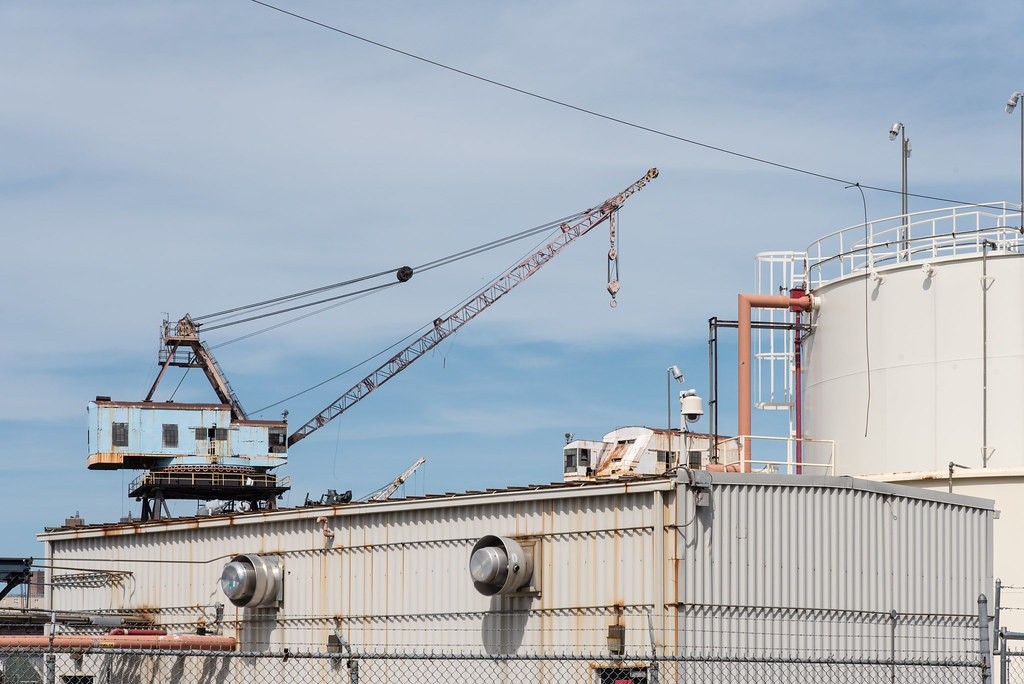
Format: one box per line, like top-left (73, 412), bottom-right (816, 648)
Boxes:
top-left (681, 396), bottom-right (704, 423)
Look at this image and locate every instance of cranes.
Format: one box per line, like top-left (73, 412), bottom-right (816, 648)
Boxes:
top-left (159, 164), bottom-right (661, 457)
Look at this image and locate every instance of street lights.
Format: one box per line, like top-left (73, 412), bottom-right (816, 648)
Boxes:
top-left (1005, 91), bottom-right (1024, 232)
top-left (889, 122), bottom-right (911, 256)
